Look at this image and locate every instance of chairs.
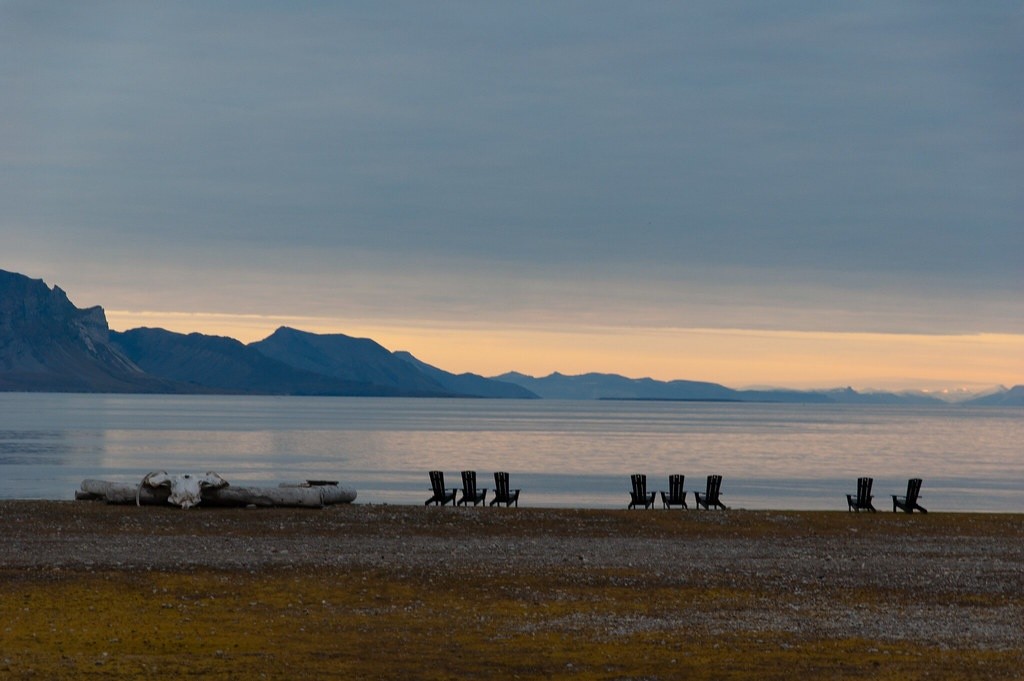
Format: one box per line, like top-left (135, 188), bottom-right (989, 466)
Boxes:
top-left (490, 471), bottom-right (520, 507)
top-left (456, 470), bottom-right (487, 507)
top-left (424, 470), bottom-right (457, 506)
top-left (661, 474), bottom-right (688, 509)
top-left (891, 477), bottom-right (928, 514)
top-left (628, 473), bottom-right (656, 510)
top-left (695, 475), bottom-right (726, 510)
top-left (845, 477), bottom-right (876, 514)
